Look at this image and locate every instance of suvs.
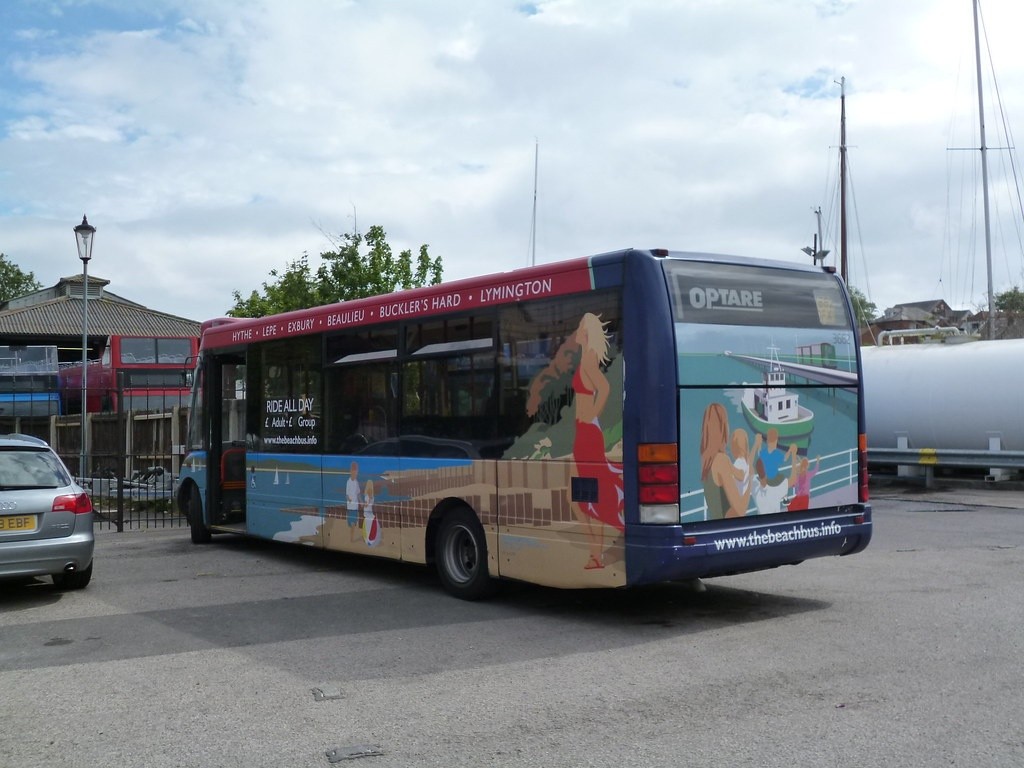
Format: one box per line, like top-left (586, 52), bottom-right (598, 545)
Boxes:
top-left (0, 432), bottom-right (95, 594)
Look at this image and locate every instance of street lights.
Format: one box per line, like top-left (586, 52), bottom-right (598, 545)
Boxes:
top-left (71, 213), bottom-right (99, 488)
top-left (802, 234), bottom-right (830, 266)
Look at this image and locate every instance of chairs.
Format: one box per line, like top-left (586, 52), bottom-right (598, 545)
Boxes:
top-left (18, 352), bottom-right (190, 371)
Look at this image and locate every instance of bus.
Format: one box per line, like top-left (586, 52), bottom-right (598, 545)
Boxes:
top-left (167, 243), bottom-right (874, 595)
